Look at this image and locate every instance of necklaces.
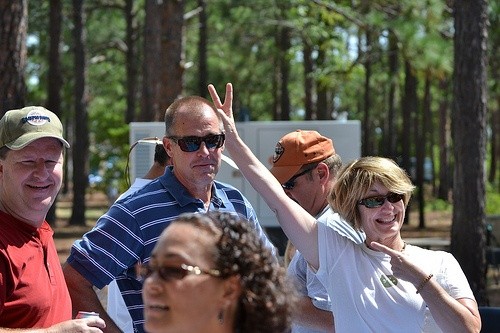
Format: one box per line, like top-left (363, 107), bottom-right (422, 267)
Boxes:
top-left (364, 239), bottom-right (406, 252)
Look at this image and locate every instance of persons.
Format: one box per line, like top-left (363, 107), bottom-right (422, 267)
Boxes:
top-left (270, 129), bottom-right (343, 333)
top-left (62, 92), bottom-right (280, 333)
top-left (208, 83), bottom-right (481, 333)
top-left (107, 144), bottom-right (173, 333)
top-left (0, 106), bottom-right (106, 333)
top-left (143, 209), bottom-right (299, 333)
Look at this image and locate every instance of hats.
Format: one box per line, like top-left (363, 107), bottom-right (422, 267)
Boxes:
top-left (0, 106), bottom-right (70, 150)
top-left (269, 130), bottom-right (335, 186)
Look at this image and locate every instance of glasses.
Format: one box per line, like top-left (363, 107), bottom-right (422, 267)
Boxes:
top-left (356, 191), bottom-right (404, 208)
top-left (170, 132), bottom-right (225, 152)
top-left (281, 162), bottom-right (330, 190)
top-left (134, 263), bottom-right (229, 283)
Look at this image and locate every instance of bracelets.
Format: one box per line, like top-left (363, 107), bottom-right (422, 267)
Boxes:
top-left (416, 274), bottom-right (434, 295)
